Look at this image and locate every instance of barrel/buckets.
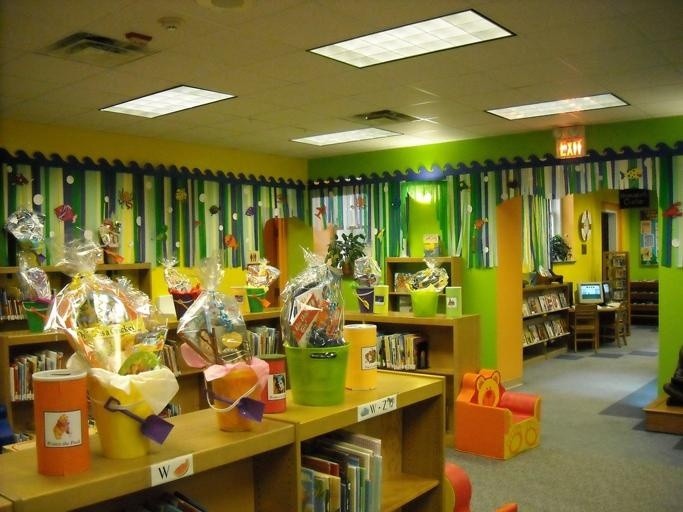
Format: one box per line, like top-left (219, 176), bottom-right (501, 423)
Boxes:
top-left (169, 290), bottom-right (194, 321)
top-left (22, 300), bottom-right (50, 335)
top-left (409, 292), bottom-right (439, 317)
top-left (284, 342), bottom-right (352, 407)
top-left (353, 287), bottom-right (374, 313)
top-left (202, 364), bottom-right (269, 433)
top-left (84, 375), bottom-right (152, 458)
top-left (247, 285), bottom-right (267, 313)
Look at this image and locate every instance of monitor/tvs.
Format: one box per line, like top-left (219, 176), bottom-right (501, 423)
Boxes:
top-left (579, 282), bottom-right (603, 305)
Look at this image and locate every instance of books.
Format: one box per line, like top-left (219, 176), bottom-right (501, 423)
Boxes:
top-left (136, 489), bottom-right (207, 512)
top-left (302, 429), bottom-right (383, 511)
top-left (1, 287), bottom-right (430, 445)
top-left (524, 254), bottom-right (630, 348)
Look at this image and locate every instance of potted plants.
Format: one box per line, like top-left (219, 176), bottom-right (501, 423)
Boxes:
top-left (322, 230), bottom-right (368, 277)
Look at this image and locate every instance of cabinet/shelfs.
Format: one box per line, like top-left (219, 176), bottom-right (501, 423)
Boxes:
top-left (0, 266), bottom-right (285, 448)
top-left (602, 251), bottom-right (632, 337)
top-left (343, 309), bottom-right (479, 381)
top-left (2, 369), bottom-right (446, 512)
top-left (630, 280), bottom-right (659, 321)
top-left (382, 255), bottom-right (462, 312)
top-left (522, 282), bottom-right (573, 360)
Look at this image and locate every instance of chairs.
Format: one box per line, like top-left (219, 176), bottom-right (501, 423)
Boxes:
top-left (443, 463), bottom-right (519, 512)
top-left (570, 301), bottom-right (630, 352)
top-left (455, 370), bottom-right (541, 457)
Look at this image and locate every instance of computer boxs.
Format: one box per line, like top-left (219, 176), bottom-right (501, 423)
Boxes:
top-left (602, 282), bottom-right (612, 304)
